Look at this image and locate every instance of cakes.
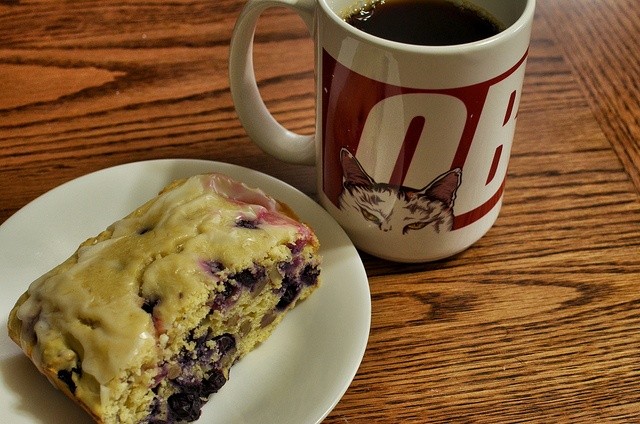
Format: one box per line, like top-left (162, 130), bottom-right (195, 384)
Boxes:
top-left (7, 172), bottom-right (323, 424)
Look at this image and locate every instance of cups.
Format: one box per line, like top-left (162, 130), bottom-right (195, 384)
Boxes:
top-left (228, 1), bottom-right (536, 263)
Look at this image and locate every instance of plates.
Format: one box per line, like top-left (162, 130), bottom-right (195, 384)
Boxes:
top-left (0, 158), bottom-right (373, 424)
